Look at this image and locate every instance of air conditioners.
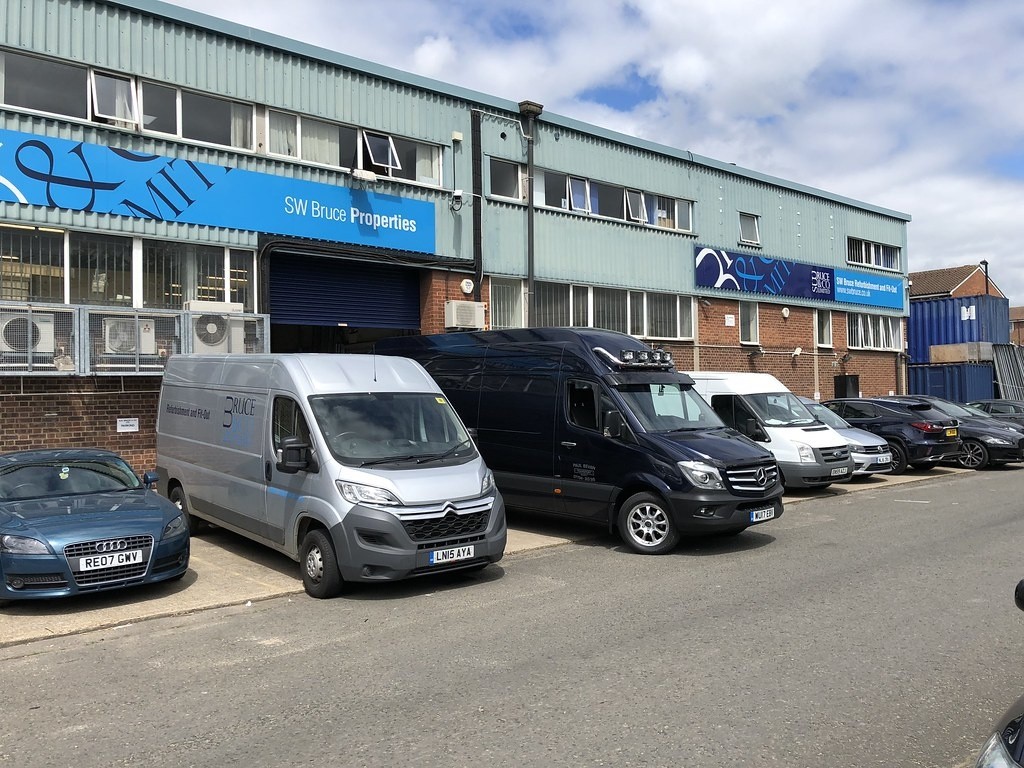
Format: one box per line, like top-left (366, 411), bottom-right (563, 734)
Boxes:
top-left (0, 312), bottom-right (55, 352)
top-left (444, 300), bottom-right (485, 329)
top-left (183, 300), bottom-right (244, 354)
top-left (102, 318), bottom-right (155, 354)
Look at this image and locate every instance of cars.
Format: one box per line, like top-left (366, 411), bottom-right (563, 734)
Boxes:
top-left (892, 395), bottom-right (1024, 469)
top-left (0, 446), bottom-right (191, 602)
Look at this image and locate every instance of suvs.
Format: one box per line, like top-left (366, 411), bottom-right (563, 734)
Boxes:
top-left (819, 398), bottom-right (963, 473)
top-left (768, 395), bottom-right (893, 477)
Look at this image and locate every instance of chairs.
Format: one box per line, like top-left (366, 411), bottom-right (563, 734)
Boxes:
top-left (571, 389), bottom-right (605, 432)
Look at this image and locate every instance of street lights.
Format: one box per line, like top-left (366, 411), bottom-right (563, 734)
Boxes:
top-left (980, 260), bottom-right (989, 294)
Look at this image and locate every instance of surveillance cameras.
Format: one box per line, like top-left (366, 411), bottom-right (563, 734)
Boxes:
top-left (794, 348), bottom-right (802, 356)
top-left (909, 281), bottom-right (912, 286)
top-left (453, 189), bottom-right (463, 202)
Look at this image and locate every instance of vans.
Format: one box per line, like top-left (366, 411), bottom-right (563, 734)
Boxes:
top-left (376, 325), bottom-right (784, 553)
top-left (653, 372), bottom-right (856, 493)
top-left (155, 354), bottom-right (506, 599)
